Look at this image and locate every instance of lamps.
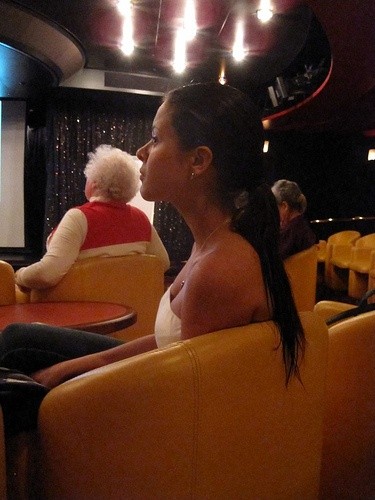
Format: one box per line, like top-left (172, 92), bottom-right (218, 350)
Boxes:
top-left (367, 149), bottom-right (375, 161)
top-left (262, 139), bottom-right (272, 154)
top-left (114, 0), bottom-right (281, 75)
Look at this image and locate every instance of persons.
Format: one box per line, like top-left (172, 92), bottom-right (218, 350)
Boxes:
top-left (13, 144), bottom-right (171, 295)
top-left (272, 180), bottom-right (313, 261)
top-left (10, 82), bottom-right (305, 500)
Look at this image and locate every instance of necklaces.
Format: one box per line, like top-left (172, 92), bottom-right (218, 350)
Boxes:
top-left (181, 218), bottom-right (232, 288)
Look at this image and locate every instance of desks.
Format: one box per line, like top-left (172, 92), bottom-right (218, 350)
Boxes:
top-left (0, 301), bottom-right (140, 349)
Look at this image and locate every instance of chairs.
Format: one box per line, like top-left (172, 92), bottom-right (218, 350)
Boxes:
top-left (316, 229), bottom-right (375, 304)
top-left (272, 243), bottom-right (317, 310)
top-left (29, 254), bottom-right (166, 343)
top-left (0, 260), bottom-right (19, 305)
top-left (312, 299), bottom-right (375, 500)
top-left (36, 308), bottom-right (328, 500)
